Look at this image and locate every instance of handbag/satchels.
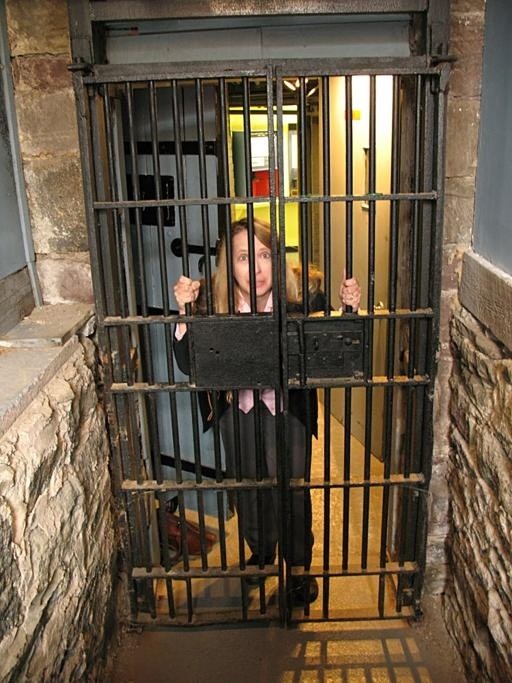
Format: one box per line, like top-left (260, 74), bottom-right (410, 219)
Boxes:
top-left (154, 507), bottom-right (220, 562)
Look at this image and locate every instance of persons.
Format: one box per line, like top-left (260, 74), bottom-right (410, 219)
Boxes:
top-left (170, 217), bottom-right (361, 607)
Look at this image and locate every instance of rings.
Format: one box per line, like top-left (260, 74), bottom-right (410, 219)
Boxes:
top-left (350, 293), bottom-right (354, 301)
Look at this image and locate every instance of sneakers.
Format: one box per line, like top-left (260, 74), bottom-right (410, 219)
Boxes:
top-left (287, 572), bottom-right (318, 609)
top-left (244, 550), bottom-right (278, 586)
